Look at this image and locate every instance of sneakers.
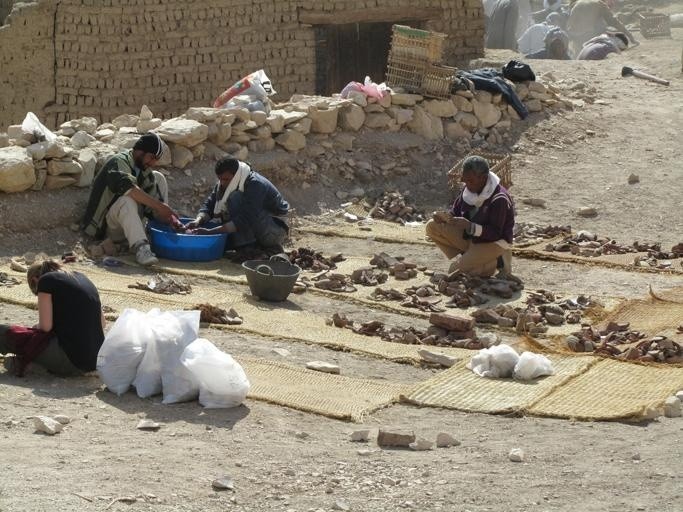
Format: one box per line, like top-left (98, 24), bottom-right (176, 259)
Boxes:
top-left (4, 356), bottom-right (48, 378)
top-left (135, 244), bottom-right (159, 266)
top-left (495, 249), bottom-right (512, 278)
top-left (231, 254), bottom-right (270, 264)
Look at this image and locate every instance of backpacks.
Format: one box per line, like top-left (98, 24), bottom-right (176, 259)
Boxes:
top-left (502, 60), bottom-right (535, 82)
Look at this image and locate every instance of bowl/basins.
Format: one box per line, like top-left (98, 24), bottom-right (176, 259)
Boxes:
top-left (145, 217), bottom-right (229, 262)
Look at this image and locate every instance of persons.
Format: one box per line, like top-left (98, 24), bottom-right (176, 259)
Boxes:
top-left (180, 156), bottom-right (291, 263)
top-left (0, 259), bottom-right (104, 377)
top-left (427, 155), bottom-right (512, 282)
top-left (484, 0), bottom-right (641, 60)
top-left (84, 132), bottom-right (187, 267)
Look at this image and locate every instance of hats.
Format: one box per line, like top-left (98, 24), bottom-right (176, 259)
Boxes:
top-left (132, 132), bottom-right (164, 160)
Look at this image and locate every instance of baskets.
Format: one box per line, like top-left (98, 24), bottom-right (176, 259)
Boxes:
top-left (446, 149), bottom-right (512, 193)
top-left (388, 56), bottom-right (458, 101)
top-left (388, 24), bottom-right (449, 65)
